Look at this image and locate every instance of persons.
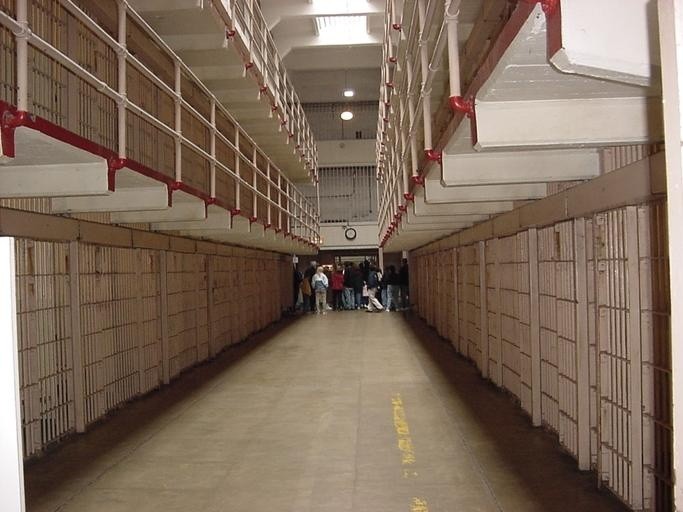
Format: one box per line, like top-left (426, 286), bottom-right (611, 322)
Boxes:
top-left (291, 256), bottom-right (409, 315)
